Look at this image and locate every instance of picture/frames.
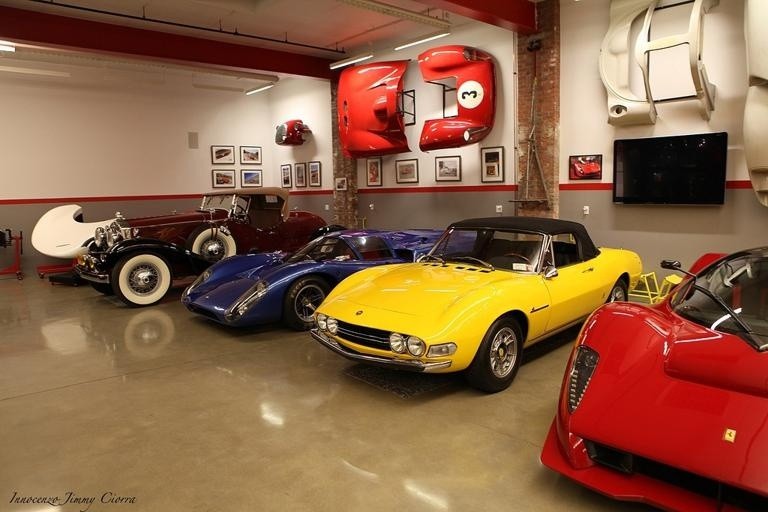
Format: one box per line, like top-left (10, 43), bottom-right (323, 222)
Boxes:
top-left (567, 153), bottom-right (602, 181)
top-left (364, 156), bottom-right (385, 187)
top-left (394, 157), bottom-right (419, 185)
top-left (480, 145), bottom-right (506, 183)
top-left (209, 141), bottom-right (324, 190)
top-left (434, 154), bottom-right (463, 182)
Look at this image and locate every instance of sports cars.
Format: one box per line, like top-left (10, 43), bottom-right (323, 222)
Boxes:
top-left (180, 229), bottom-right (479, 333)
top-left (541, 247), bottom-right (768, 511)
top-left (571, 155), bottom-right (601, 179)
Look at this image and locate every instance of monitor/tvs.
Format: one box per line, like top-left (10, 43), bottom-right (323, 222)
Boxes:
top-left (611, 130), bottom-right (728, 206)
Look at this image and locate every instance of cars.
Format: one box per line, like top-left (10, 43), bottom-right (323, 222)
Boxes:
top-left (75, 185), bottom-right (326, 310)
top-left (83, 284), bottom-right (243, 384)
top-left (312, 216), bottom-right (642, 394)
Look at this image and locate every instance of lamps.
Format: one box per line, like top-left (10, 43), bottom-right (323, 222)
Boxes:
top-left (393, 7), bottom-right (454, 51)
top-left (241, 81), bottom-right (275, 97)
top-left (328, 30), bottom-right (377, 70)
